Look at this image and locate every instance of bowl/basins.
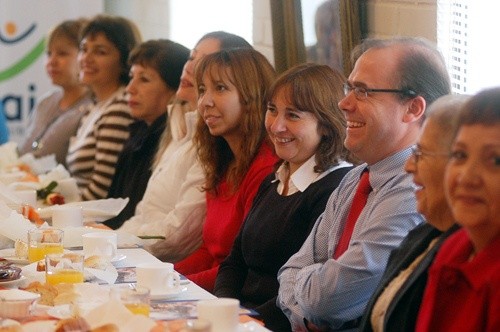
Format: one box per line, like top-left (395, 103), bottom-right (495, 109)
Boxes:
top-left (0, 290), bottom-right (40, 317)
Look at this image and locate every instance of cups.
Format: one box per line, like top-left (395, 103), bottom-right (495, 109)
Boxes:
top-left (28, 229), bottom-right (64, 263)
top-left (47, 256), bottom-right (85, 286)
top-left (52, 207), bottom-right (83, 229)
top-left (123, 288), bottom-right (151, 317)
top-left (199, 298), bottom-right (239, 332)
top-left (136, 262), bottom-right (180, 292)
top-left (83, 233), bottom-right (117, 259)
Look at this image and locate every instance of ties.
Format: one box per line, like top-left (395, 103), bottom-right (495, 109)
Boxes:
top-left (370, 236), bottom-right (444, 332)
top-left (333, 172), bottom-right (372, 260)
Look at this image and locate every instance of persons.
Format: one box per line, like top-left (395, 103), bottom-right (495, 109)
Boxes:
top-left (17, 18), bottom-right (98, 169)
top-left (67, 14), bottom-right (144, 202)
top-left (100, 31), bottom-right (500, 332)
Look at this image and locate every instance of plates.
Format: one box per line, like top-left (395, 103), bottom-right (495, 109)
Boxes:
top-left (1, 249), bottom-right (27, 264)
top-left (0, 275), bottom-right (26, 285)
top-left (47, 305), bottom-right (91, 318)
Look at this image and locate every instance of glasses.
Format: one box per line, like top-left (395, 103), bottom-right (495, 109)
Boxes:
top-left (412, 143), bottom-right (451, 160)
top-left (342, 82), bottom-right (419, 101)
